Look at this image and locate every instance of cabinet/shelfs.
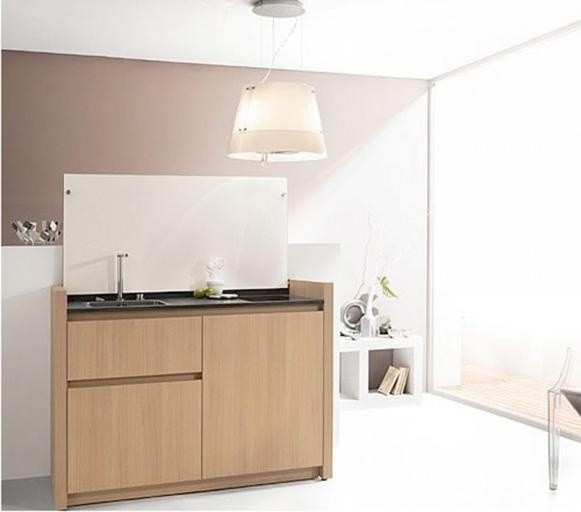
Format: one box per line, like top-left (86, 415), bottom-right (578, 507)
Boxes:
top-left (64, 310), bottom-right (323, 493)
top-left (340, 332), bottom-right (425, 400)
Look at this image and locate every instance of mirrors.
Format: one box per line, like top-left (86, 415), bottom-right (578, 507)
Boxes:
top-left (63, 172), bottom-right (288, 294)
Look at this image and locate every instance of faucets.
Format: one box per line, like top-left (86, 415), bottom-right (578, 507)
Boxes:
top-left (116, 253), bottom-right (130, 301)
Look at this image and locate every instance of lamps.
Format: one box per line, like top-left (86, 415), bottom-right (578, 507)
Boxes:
top-left (226, 0), bottom-right (327, 169)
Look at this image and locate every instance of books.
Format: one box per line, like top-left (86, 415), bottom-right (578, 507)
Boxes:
top-left (376, 362), bottom-right (411, 396)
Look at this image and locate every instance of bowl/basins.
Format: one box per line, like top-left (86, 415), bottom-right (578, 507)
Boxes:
top-left (10, 218), bottom-right (62, 245)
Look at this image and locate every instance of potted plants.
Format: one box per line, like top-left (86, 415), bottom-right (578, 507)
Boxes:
top-left (362, 275), bottom-right (397, 337)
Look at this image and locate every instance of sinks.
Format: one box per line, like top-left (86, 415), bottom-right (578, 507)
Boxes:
top-left (87, 300), bottom-right (166, 306)
